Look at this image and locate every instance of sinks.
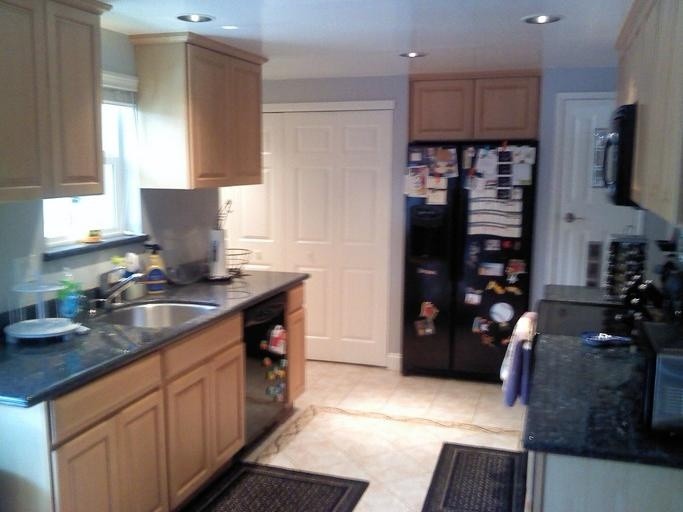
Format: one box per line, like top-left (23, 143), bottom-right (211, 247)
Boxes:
top-left (95, 300), bottom-right (219, 329)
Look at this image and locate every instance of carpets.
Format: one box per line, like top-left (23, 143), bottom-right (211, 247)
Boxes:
top-left (179, 459), bottom-right (369, 511)
top-left (421, 442), bottom-right (529, 512)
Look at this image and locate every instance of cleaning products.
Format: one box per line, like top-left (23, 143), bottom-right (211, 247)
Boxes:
top-left (144, 242), bottom-right (165, 282)
top-left (148, 284), bottom-right (167, 295)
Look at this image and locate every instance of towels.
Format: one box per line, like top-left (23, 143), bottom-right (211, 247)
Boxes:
top-left (499, 311), bottom-right (538, 406)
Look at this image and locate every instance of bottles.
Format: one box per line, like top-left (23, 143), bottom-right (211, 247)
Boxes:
top-left (56, 267), bottom-right (78, 317)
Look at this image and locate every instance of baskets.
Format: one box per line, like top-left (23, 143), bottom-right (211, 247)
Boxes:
top-left (214, 247), bottom-right (252, 275)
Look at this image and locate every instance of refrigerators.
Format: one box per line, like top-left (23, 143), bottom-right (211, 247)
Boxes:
top-left (403, 141), bottom-right (536, 381)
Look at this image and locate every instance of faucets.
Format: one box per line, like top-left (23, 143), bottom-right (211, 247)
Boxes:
top-left (99, 266), bottom-right (145, 311)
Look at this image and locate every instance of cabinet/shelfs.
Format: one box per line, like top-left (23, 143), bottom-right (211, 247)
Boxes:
top-left (168, 312), bottom-right (247, 512)
top-left (130, 32), bottom-right (269, 190)
top-left (0, 0), bottom-right (112, 202)
top-left (409, 74), bottom-right (540, 143)
top-left (0, 346), bottom-right (171, 512)
top-left (616, 1), bottom-right (683, 226)
